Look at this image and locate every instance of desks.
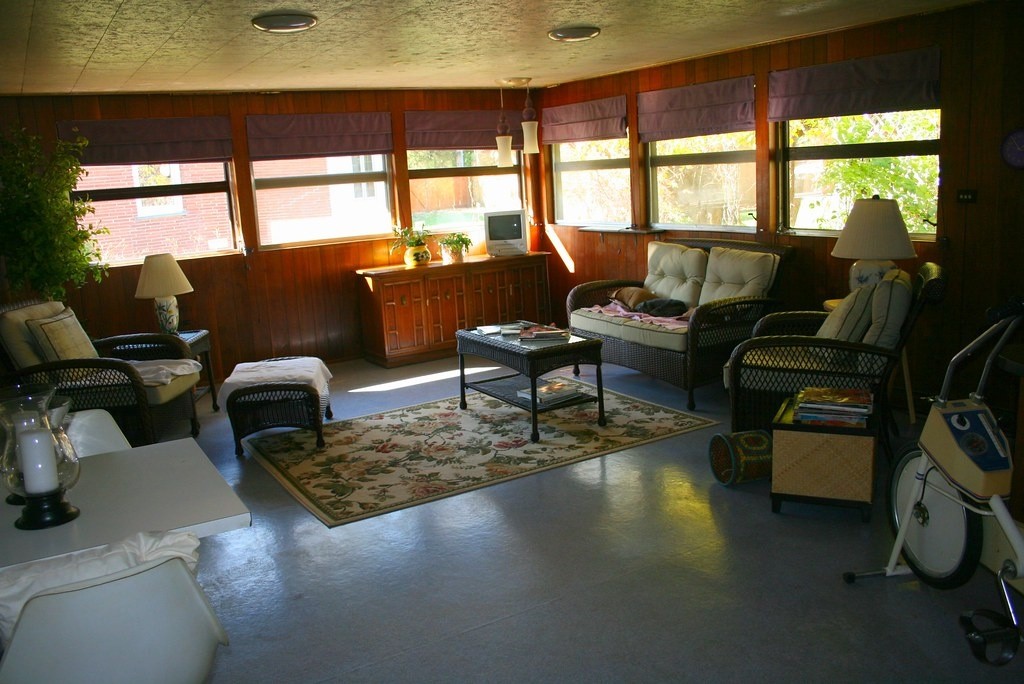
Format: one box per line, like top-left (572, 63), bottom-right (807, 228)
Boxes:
top-left (0, 437), bottom-right (254, 567)
top-left (216, 356), bottom-right (333, 419)
top-left (455, 319), bottom-right (606, 443)
top-left (113, 330), bottom-right (220, 436)
top-left (823, 261), bottom-right (917, 425)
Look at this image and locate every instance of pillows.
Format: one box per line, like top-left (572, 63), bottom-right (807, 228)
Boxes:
top-left (0, 530), bottom-right (199, 653)
top-left (606, 287), bottom-right (659, 311)
top-left (25, 306), bottom-right (102, 383)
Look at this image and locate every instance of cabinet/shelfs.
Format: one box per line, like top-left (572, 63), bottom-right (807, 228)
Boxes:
top-left (349, 252), bottom-right (552, 369)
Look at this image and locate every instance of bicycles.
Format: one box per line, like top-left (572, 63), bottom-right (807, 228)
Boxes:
top-left (842, 317), bottom-right (1024, 671)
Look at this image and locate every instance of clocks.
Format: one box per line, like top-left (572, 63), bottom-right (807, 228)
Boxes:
top-left (1000, 131), bottom-right (1024, 168)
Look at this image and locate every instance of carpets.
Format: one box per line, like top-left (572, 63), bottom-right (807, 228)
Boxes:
top-left (241, 376), bottom-right (720, 530)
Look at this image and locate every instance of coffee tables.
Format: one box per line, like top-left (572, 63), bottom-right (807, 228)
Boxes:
top-left (770, 398), bottom-right (880, 522)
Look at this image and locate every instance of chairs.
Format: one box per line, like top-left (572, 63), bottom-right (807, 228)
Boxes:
top-left (60, 408), bottom-right (133, 460)
top-left (0, 556), bottom-right (229, 684)
top-left (227, 382), bottom-right (325, 457)
top-left (722, 261), bottom-right (949, 468)
top-left (0, 300), bottom-right (201, 439)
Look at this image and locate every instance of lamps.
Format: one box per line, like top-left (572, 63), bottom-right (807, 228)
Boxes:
top-left (491, 73), bottom-right (546, 169)
top-left (134, 253), bottom-right (195, 333)
top-left (830, 194), bottom-right (918, 294)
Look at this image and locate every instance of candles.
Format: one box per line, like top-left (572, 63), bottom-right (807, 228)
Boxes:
top-left (22, 424), bottom-right (60, 494)
top-left (13, 407), bottom-right (39, 472)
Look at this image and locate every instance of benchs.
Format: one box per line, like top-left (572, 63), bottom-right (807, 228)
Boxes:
top-left (566, 239), bottom-right (793, 411)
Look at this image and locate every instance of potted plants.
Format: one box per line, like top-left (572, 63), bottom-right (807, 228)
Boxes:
top-left (437, 232), bottom-right (472, 263)
top-left (388, 222), bottom-right (434, 266)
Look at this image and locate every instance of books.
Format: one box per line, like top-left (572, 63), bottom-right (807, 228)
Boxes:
top-left (517, 381), bottom-right (577, 404)
top-left (477, 323), bottom-right (522, 336)
top-left (792, 387), bottom-right (873, 427)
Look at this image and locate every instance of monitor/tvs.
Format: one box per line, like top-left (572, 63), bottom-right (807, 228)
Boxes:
top-left (484, 209), bottom-right (527, 256)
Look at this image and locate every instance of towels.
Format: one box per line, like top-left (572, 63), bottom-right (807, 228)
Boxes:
top-left (53, 357), bottom-right (203, 389)
top-left (216, 356), bottom-right (333, 413)
